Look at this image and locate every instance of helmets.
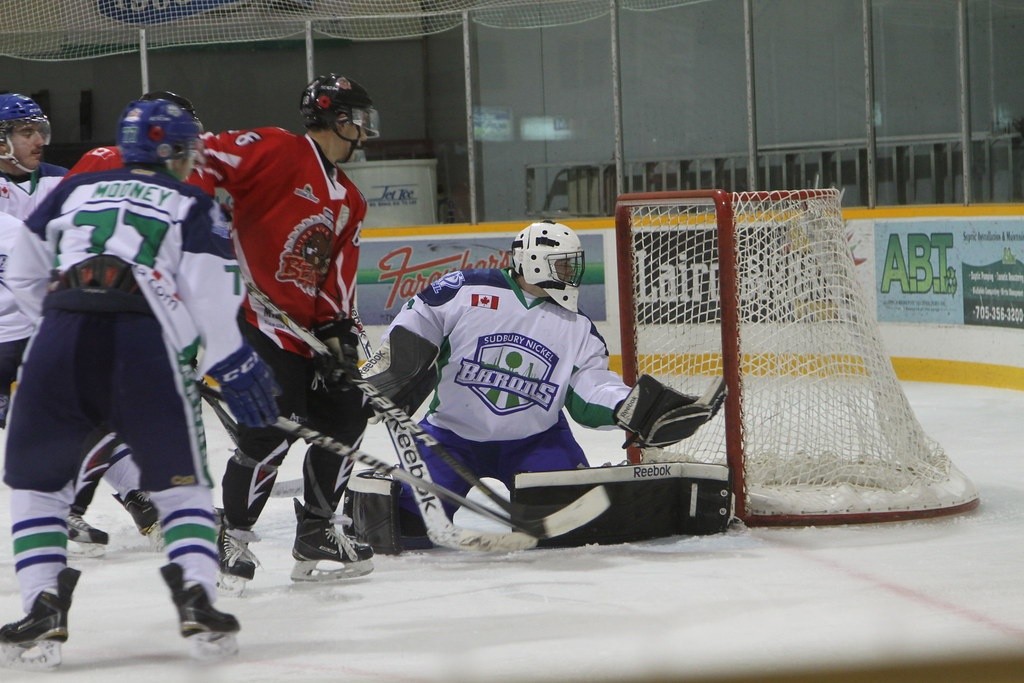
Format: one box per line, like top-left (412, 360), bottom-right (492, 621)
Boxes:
top-left (138, 92), bottom-right (197, 119)
top-left (0, 93), bottom-right (43, 142)
top-left (118, 99), bottom-right (199, 162)
top-left (301, 73), bottom-right (372, 126)
top-left (512, 220), bottom-right (585, 314)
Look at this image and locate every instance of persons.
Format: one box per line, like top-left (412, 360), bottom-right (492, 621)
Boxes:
top-left (0, 73), bottom-right (379, 672)
top-left (341, 221), bottom-right (746, 556)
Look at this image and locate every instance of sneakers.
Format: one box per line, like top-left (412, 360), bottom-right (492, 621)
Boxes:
top-left (0, 568), bottom-right (81, 670)
top-left (217, 508), bottom-right (259, 595)
top-left (161, 562), bottom-right (240, 658)
top-left (67, 504), bottom-right (109, 559)
top-left (113, 489), bottom-right (164, 550)
top-left (291, 496), bottom-right (374, 581)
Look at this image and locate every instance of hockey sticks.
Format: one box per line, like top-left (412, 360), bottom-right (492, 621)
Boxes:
top-left (243, 278), bottom-right (573, 521)
top-left (350, 306), bottom-right (538, 553)
top-left (198, 373), bottom-right (614, 541)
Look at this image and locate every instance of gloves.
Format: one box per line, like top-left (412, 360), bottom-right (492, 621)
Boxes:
top-left (315, 317), bottom-right (364, 395)
top-left (205, 337), bottom-right (281, 427)
